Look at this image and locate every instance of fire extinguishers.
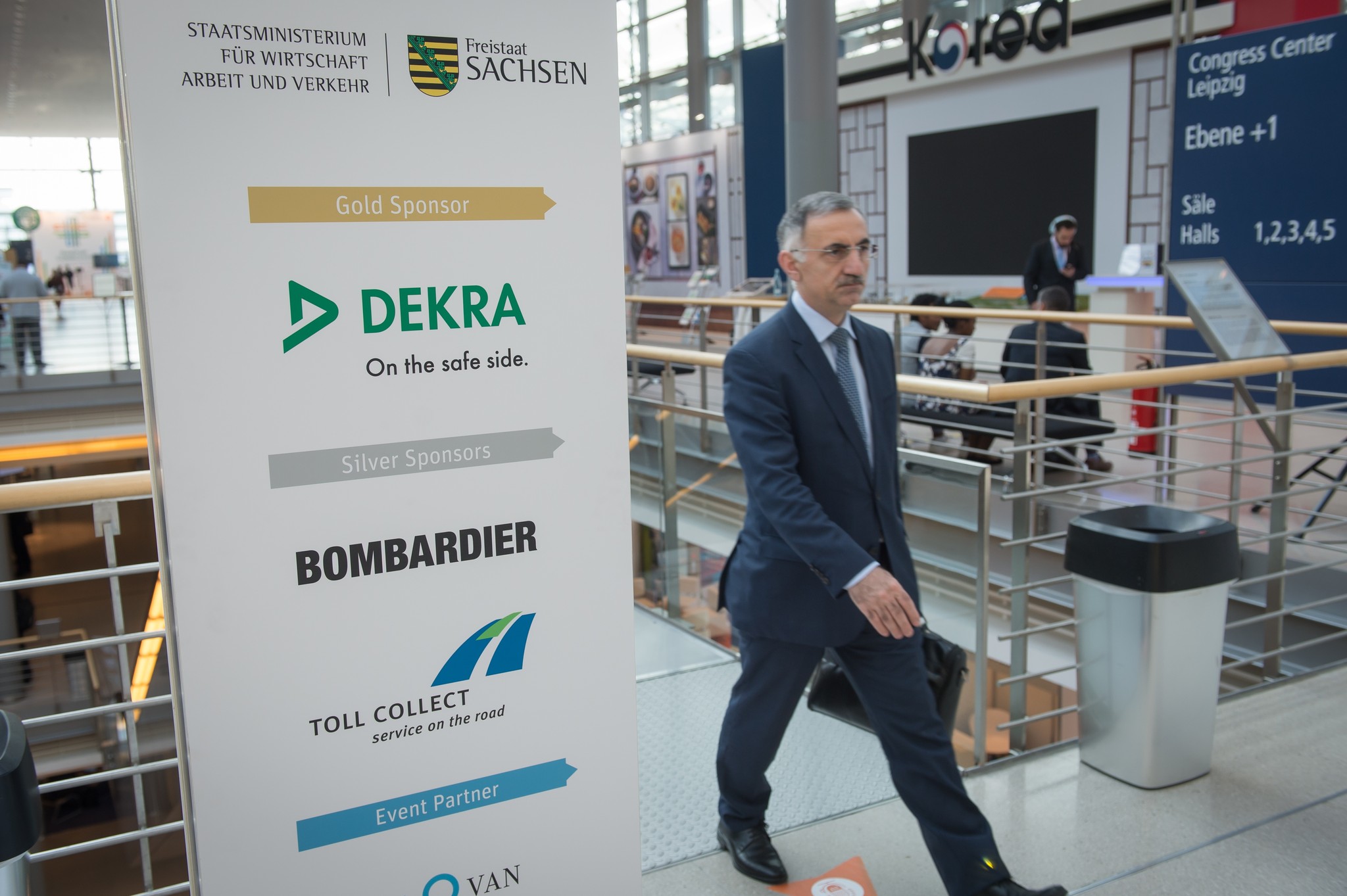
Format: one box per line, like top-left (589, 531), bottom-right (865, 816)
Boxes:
top-left (1128, 356), bottom-right (1159, 457)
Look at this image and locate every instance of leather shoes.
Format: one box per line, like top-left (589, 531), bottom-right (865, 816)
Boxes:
top-left (978, 878), bottom-right (1068, 896)
top-left (715, 817), bottom-right (789, 883)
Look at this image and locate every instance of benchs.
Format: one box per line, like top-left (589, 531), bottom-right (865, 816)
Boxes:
top-left (899, 398), bottom-right (1115, 477)
top-left (627, 357), bottom-right (696, 406)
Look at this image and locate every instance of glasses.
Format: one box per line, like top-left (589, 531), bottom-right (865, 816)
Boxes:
top-left (790, 245), bottom-right (880, 259)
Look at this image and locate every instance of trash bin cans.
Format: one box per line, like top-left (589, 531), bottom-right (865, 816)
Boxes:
top-left (1064, 503), bottom-right (1239, 790)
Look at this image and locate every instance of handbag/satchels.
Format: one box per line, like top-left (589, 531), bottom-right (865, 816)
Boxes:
top-left (807, 622), bottom-right (969, 744)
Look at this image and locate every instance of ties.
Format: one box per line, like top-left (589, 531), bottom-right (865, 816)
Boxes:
top-left (827, 327), bottom-right (870, 456)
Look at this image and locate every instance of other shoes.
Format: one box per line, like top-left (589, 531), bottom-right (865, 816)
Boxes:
top-left (1086, 455), bottom-right (1112, 473)
top-left (968, 452), bottom-right (1003, 464)
top-left (934, 434), bottom-right (948, 443)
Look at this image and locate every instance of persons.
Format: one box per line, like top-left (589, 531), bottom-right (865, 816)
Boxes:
top-left (890, 292), bottom-right (962, 455)
top-left (1025, 214), bottom-right (1087, 314)
top-left (1000, 287), bottom-right (1117, 472)
top-left (915, 299), bottom-right (1004, 465)
top-left (2, 251), bottom-right (55, 369)
top-left (47, 261), bottom-right (72, 321)
top-left (710, 194), bottom-right (1072, 896)
